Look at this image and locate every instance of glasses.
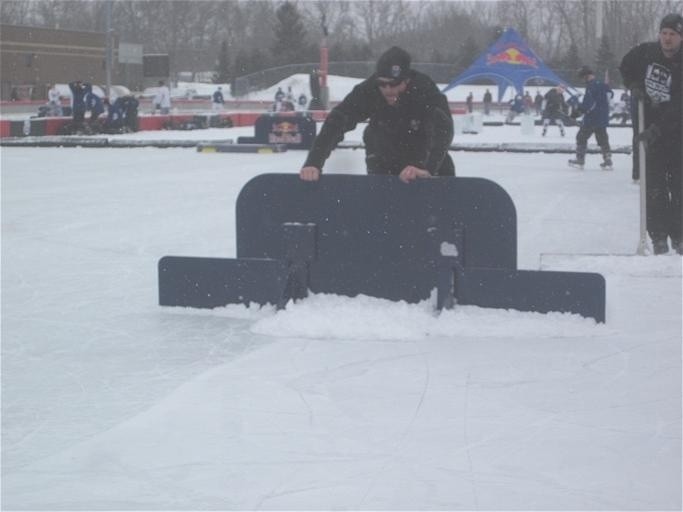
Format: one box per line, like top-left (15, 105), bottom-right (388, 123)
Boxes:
top-left (374, 78), bottom-right (401, 88)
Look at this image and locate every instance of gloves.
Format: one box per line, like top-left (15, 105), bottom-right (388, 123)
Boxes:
top-left (634, 123), bottom-right (662, 153)
top-left (632, 88), bottom-right (652, 105)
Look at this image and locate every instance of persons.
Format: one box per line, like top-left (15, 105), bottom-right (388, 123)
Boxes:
top-left (271, 86), bottom-right (296, 113)
top-left (300, 47), bottom-right (456, 184)
top-left (620, 13), bottom-right (682, 254)
top-left (466, 92), bottom-right (476, 111)
top-left (211, 87), bottom-right (225, 113)
top-left (45, 83), bottom-right (64, 116)
top-left (507, 85), bottom-right (632, 136)
top-left (483, 89), bottom-right (493, 112)
top-left (154, 81), bottom-right (171, 115)
top-left (68, 80), bottom-right (140, 137)
top-left (567, 65), bottom-right (614, 169)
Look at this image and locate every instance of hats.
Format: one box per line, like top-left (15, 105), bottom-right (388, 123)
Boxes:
top-left (377, 46), bottom-right (410, 78)
top-left (660, 14), bottom-right (683, 36)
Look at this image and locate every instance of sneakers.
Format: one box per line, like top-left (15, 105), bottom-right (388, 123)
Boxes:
top-left (651, 232), bottom-right (668, 255)
top-left (671, 239), bottom-right (683, 255)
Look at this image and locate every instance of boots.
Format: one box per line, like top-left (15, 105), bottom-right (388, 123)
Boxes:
top-left (600, 152), bottom-right (612, 167)
top-left (568, 152), bottom-right (585, 164)
